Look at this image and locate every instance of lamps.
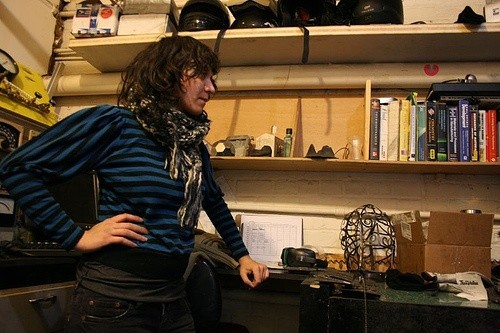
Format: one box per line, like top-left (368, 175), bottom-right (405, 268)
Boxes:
top-left (0, 48), bottom-right (19, 83)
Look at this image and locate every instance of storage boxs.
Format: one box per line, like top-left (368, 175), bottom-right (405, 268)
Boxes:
top-left (119, 0), bottom-right (180, 30)
top-left (71, 1), bottom-right (123, 39)
top-left (117, 13), bottom-right (177, 36)
top-left (389, 209), bottom-right (494, 282)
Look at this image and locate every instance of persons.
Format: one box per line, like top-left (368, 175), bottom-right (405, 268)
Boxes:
top-left (1, 34), bottom-right (270, 332)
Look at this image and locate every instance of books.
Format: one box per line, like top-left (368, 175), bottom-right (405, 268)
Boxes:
top-left (370, 81), bottom-right (500, 162)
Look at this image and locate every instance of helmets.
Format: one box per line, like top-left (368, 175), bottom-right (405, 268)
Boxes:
top-left (337, 0), bottom-right (404, 25)
top-left (227, 0), bottom-right (281, 28)
top-left (178, 0), bottom-right (230, 31)
top-left (277, 0), bottom-right (338, 27)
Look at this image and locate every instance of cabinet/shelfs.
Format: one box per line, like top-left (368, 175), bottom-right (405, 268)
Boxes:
top-left (69, 0), bottom-right (500, 177)
